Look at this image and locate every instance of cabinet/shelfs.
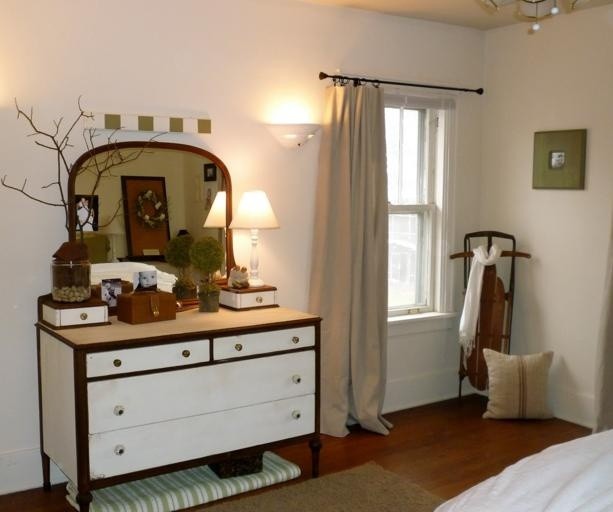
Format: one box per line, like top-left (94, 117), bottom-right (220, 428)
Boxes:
top-left (34, 305), bottom-right (325, 512)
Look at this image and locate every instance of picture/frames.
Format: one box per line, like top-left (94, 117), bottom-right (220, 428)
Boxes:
top-left (73, 194), bottom-right (100, 235)
top-left (203, 163), bottom-right (218, 182)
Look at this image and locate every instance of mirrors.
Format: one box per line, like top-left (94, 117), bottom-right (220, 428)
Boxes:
top-left (66, 139), bottom-right (239, 317)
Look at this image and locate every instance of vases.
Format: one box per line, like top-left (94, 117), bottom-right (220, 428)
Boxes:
top-left (50, 239), bottom-right (91, 303)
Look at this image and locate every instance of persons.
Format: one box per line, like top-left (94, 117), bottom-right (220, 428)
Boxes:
top-left (104, 283), bottom-right (117, 304)
top-left (134, 271), bottom-right (157, 292)
top-left (75, 198), bottom-right (94, 231)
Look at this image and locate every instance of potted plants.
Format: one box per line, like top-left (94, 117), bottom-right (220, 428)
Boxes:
top-left (188, 235), bottom-right (226, 312)
top-left (163, 234), bottom-right (199, 300)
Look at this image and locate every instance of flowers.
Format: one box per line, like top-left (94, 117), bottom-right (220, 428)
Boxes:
top-left (134, 190), bottom-right (169, 230)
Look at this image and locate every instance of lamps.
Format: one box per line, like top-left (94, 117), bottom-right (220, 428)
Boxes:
top-left (230, 190), bottom-right (280, 286)
top-left (202, 191), bottom-right (226, 278)
top-left (479, 0), bottom-right (592, 30)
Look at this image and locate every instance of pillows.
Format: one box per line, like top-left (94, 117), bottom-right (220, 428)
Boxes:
top-left (480, 347), bottom-right (558, 420)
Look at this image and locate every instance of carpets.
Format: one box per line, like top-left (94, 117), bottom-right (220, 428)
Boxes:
top-left (193, 459), bottom-right (448, 511)
